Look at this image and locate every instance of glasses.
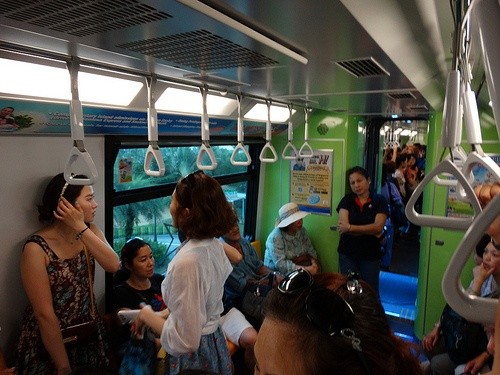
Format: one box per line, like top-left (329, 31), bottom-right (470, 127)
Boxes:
top-left (181, 170), bottom-right (206, 185)
top-left (125, 237), bottom-right (144, 245)
top-left (277, 267), bottom-right (355, 332)
top-left (347, 269), bottom-right (363, 296)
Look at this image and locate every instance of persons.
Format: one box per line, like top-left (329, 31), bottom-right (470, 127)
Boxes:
top-left (337, 167), bottom-right (388, 290)
top-left (16, 173), bottom-right (120, 375)
top-left (113, 236), bottom-right (167, 375)
top-left (0, 107), bottom-right (14, 126)
top-left (381, 141), bottom-right (427, 265)
top-left (254, 268), bottom-right (424, 375)
top-left (219, 215), bottom-right (283, 318)
top-left (221, 275), bottom-right (257, 375)
top-left (264, 203), bottom-right (318, 278)
top-left (423, 180), bottom-right (499, 375)
top-left (132, 172), bottom-right (237, 375)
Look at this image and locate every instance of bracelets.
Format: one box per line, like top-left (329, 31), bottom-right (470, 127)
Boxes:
top-left (75, 227), bottom-right (88, 239)
top-left (348, 225), bottom-right (351, 232)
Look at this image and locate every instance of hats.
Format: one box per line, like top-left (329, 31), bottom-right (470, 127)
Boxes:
top-left (274, 202), bottom-right (311, 228)
top-left (475, 234), bottom-right (500, 257)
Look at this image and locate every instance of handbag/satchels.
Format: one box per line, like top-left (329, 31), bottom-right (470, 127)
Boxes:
top-left (60, 314), bottom-right (101, 352)
top-left (391, 209), bottom-right (408, 228)
top-left (441, 301), bottom-right (490, 366)
